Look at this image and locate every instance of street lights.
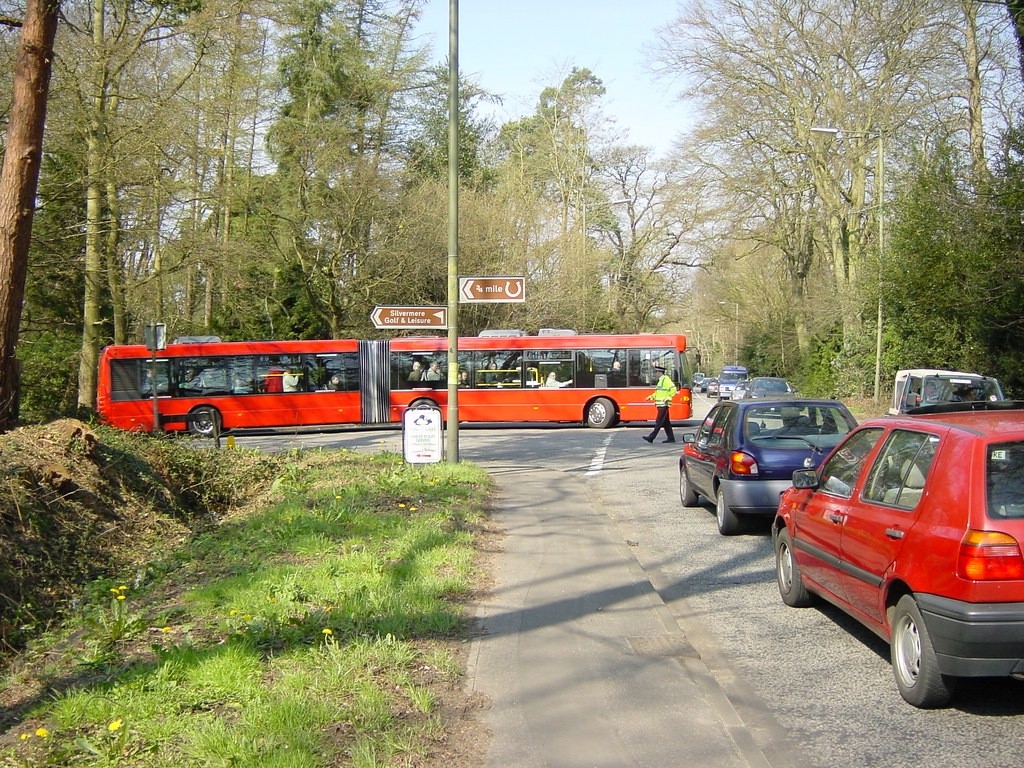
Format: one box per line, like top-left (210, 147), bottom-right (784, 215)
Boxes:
top-left (583, 199), bottom-right (629, 318)
top-left (686, 330), bottom-right (700, 373)
top-left (811, 127), bottom-right (883, 400)
top-left (719, 301), bottom-right (739, 366)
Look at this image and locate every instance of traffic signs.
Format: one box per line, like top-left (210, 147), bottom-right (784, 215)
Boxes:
top-left (457, 276), bottom-right (525, 302)
top-left (371, 306), bottom-right (448, 330)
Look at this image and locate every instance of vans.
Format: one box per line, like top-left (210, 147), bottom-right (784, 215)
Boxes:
top-left (888, 368), bottom-right (1004, 417)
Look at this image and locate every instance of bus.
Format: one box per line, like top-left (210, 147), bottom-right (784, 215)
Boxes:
top-left (96, 327), bottom-right (704, 437)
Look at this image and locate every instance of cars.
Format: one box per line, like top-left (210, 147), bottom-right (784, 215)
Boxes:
top-left (691, 373), bottom-right (705, 385)
top-left (772, 401), bottom-right (1024, 710)
top-left (701, 378), bottom-right (711, 393)
top-left (728, 380), bottom-right (751, 401)
top-left (743, 377), bottom-right (798, 399)
top-left (679, 398), bottom-right (873, 535)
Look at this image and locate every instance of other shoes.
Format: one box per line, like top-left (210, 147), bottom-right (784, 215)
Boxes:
top-left (642, 436), bottom-right (653, 443)
top-left (662, 439), bottom-right (675, 443)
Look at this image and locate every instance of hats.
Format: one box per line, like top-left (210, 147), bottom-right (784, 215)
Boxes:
top-left (653, 366), bottom-right (665, 372)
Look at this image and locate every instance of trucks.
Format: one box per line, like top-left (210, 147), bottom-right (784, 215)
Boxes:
top-left (717, 366), bottom-right (749, 401)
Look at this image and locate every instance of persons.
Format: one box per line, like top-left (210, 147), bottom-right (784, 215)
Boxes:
top-left (282, 368), bottom-right (300, 392)
top-left (142, 369), bottom-right (162, 393)
top-left (328, 376), bottom-right (339, 391)
top-left (407, 362), bottom-right (421, 381)
top-left (961, 389), bottom-right (976, 401)
top-left (458, 360), bottom-right (520, 388)
top-left (264, 366), bottom-right (283, 393)
top-left (427, 362), bottom-right (447, 381)
top-left (611, 361), bottom-right (621, 372)
top-left (184, 367), bottom-right (264, 395)
top-left (545, 372), bottom-right (572, 387)
top-left (641, 366), bottom-right (678, 444)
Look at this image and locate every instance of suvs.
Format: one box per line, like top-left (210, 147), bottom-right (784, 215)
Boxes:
top-left (706, 380), bottom-right (719, 397)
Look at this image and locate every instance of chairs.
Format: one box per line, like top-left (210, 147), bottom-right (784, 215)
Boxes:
top-left (747, 422), bottom-right (761, 435)
top-left (777, 416), bottom-right (815, 436)
top-left (882, 455), bottom-right (932, 505)
